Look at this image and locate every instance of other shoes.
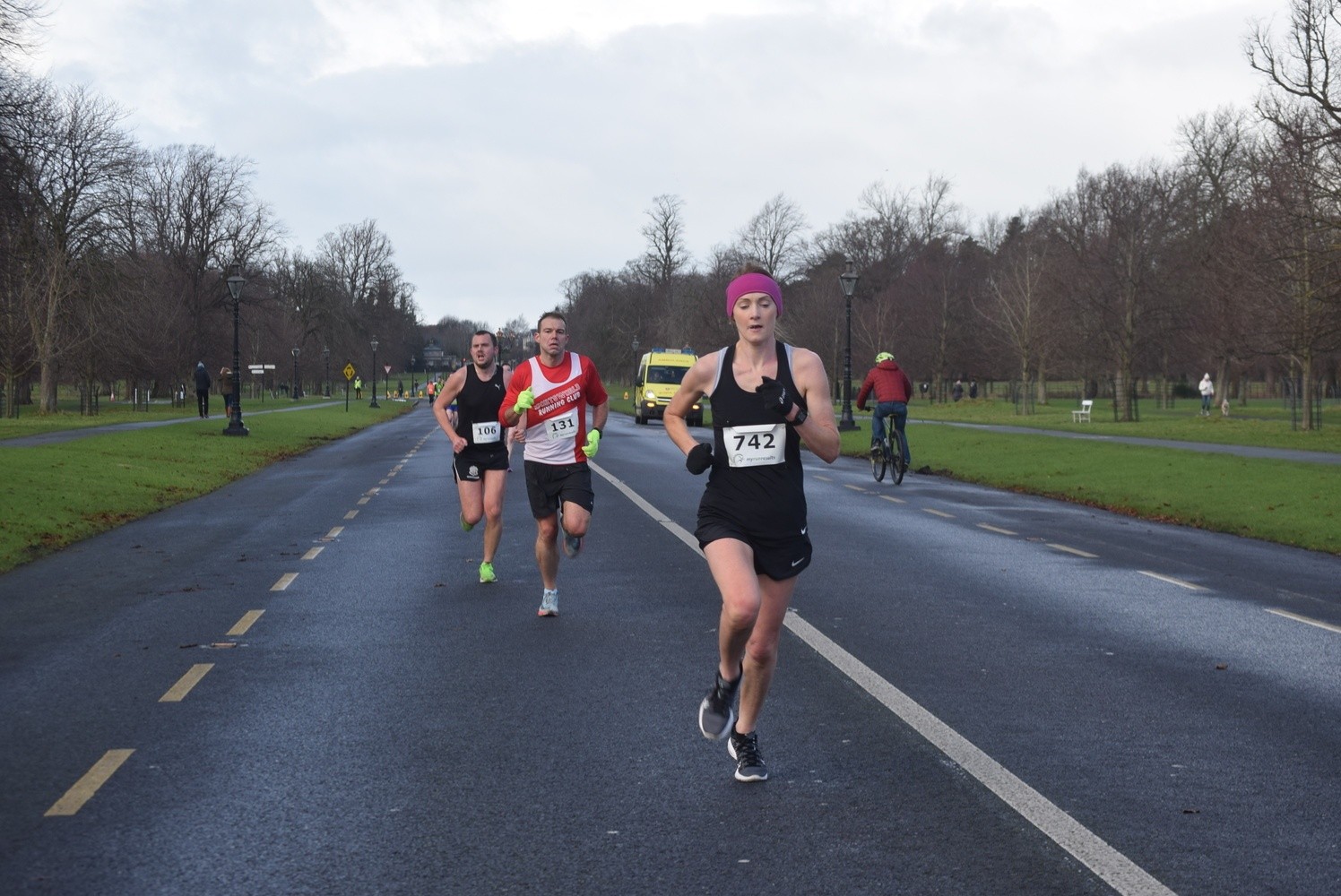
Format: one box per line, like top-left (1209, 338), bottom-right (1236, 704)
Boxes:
top-left (200, 413), bottom-right (209, 419)
top-left (870, 439), bottom-right (881, 451)
top-left (904, 459), bottom-right (909, 473)
top-left (1200, 409), bottom-right (1210, 416)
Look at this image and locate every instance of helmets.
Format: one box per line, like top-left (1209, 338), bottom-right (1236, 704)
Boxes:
top-left (875, 352), bottom-right (895, 363)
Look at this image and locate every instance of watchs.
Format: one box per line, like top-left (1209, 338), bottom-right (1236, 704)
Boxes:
top-left (593, 427), bottom-right (603, 439)
top-left (788, 407), bottom-right (807, 426)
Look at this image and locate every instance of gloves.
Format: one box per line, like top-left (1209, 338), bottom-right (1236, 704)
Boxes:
top-left (755, 376), bottom-right (792, 417)
top-left (581, 429), bottom-right (600, 458)
top-left (685, 443), bottom-right (716, 475)
top-left (514, 386), bottom-right (534, 414)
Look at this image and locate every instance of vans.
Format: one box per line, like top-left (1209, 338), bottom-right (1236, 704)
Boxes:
top-left (634, 347), bottom-right (702, 426)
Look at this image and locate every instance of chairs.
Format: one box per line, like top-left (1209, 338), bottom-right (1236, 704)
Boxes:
top-left (1073, 400), bottom-right (1094, 423)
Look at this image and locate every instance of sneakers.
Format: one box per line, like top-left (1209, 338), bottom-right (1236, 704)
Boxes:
top-left (459, 511), bottom-right (474, 531)
top-left (561, 525), bottom-right (584, 558)
top-left (727, 716), bottom-right (768, 782)
top-left (479, 561), bottom-right (497, 583)
top-left (698, 659), bottom-right (743, 741)
top-left (537, 588), bottom-right (559, 616)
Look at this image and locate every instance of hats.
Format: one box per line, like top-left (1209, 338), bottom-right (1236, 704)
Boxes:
top-left (198, 361), bottom-right (204, 368)
top-left (1205, 373), bottom-right (1210, 379)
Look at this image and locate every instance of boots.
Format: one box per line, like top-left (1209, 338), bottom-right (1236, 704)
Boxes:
top-left (225, 406), bottom-right (231, 417)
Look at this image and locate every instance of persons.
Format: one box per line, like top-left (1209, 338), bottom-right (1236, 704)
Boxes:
top-left (501, 363), bottom-right (517, 472)
top-left (110, 389), bottom-right (115, 401)
top-left (173, 383), bottom-right (186, 409)
top-left (218, 367), bottom-right (234, 417)
top-left (414, 379), bottom-right (418, 392)
top-left (918, 380), bottom-right (929, 401)
top-left (663, 266), bottom-right (842, 780)
top-left (432, 330), bottom-right (527, 582)
top-left (397, 378), bottom-right (403, 398)
top-left (968, 379), bottom-right (978, 399)
top-left (1198, 372), bottom-right (1214, 417)
top-left (354, 375), bottom-right (362, 400)
top-left (856, 351), bottom-right (911, 471)
top-left (192, 361), bottom-right (209, 419)
top-left (427, 363), bottom-right (459, 430)
top-left (499, 313), bottom-right (607, 615)
top-left (951, 379), bottom-right (963, 402)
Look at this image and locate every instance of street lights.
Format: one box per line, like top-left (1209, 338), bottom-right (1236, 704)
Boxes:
top-left (224, 259), bottom-right (249, 436)
top-left (323, 344), bottom-right (330, 398)
top-left (838, 258), bottom-right (862, 433)
top-left (291, 343), bottom-right (301, 401)
top-left (369, 334), bottom-right (380, 408)
top-left (632, 334), bottom-right (639, 390)
top-left (409, 356), bottom-right (451, 399)
top-left (384, 362), bottom-right (391, 401)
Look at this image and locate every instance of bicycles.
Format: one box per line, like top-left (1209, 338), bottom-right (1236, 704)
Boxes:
top-left (861, 405), bottom-right (907, 485)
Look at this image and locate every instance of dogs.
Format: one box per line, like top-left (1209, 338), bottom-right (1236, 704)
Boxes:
top-left (1219, 398), bottom-right (1230, 417)
